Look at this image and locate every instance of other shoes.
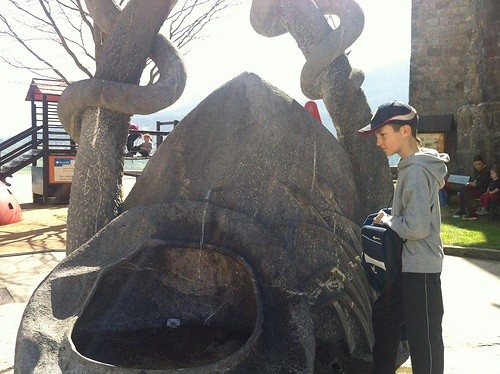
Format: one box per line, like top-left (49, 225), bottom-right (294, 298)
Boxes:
top-left (462, 214), bottom-right (477, 220)
top-left (475, 207), bottom-right (481, 213)
top-left (453, 210), bottom-right (466, 218)
top-left (478, 207), bottom-right (487, 214)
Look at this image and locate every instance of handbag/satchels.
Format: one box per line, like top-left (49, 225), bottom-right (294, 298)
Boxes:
top-left (360, 208), bottom-right (408, 297)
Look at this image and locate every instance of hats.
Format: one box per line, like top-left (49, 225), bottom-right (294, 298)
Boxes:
top-left (358, 99), bottom-right (419, 136)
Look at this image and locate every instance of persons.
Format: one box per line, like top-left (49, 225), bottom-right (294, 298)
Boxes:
top-left (125, 122), bottom-right (142, 157)
top-left (438, 173), bottom-right (451, 205)
top-left (474, 163), bottom-right (499, 216)
top-left (453, 154), bottom-right (489, 220)
top-left (125, 133), bottom-right (154, 158)
top-left (357, 97), bottom-right (449, 374)
